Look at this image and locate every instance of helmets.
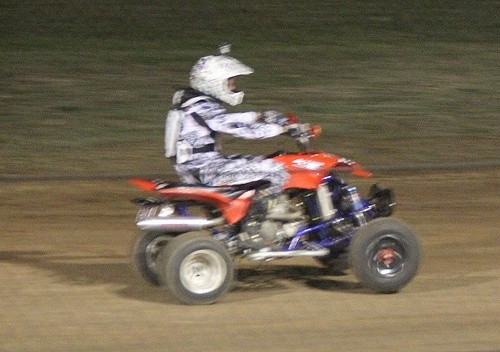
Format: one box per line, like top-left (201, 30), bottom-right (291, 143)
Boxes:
top-left (188, 54), bottom-right (255, 107)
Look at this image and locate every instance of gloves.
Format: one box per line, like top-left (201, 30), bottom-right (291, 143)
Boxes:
top-left (263, 110), bottom-right (284, 124)
top-left (287, 123), bottom-right (305, 137)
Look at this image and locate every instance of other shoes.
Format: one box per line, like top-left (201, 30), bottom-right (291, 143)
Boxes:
top-left (242, 211), bottom-right (303, 232)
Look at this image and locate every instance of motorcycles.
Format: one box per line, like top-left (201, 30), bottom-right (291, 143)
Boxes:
top-left (127, 113), bottom-right (420, 305)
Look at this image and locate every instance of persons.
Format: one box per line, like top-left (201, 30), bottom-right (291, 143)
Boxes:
top-left (164, 54), bottom-right (311, 252)
